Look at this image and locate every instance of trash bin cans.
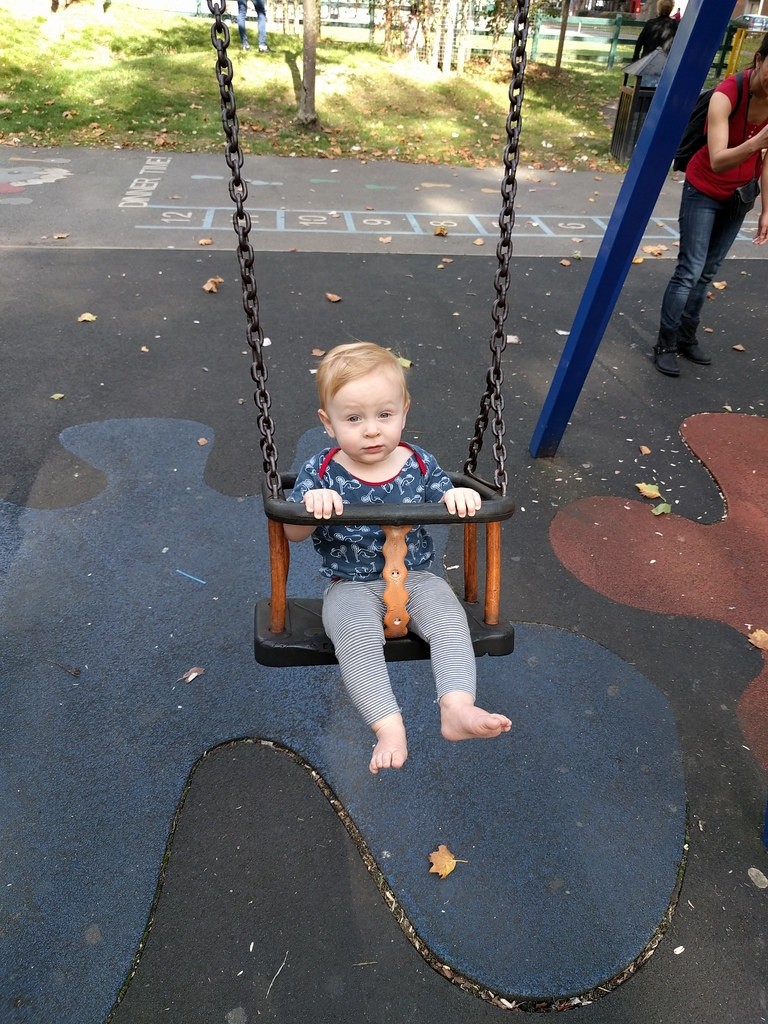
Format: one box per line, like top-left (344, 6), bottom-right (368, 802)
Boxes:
top-left (610, 47), bottom-right (670, 166)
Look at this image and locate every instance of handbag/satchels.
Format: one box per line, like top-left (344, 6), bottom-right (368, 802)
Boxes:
top-left (730, 180), bottom-right (760, 216)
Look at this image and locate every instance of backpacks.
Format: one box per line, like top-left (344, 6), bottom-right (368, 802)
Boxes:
top-left (673, 72), bottom-right (743, 173)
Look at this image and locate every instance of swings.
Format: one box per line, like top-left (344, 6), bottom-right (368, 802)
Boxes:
top-left (207, 0), bottom-right (533, 670)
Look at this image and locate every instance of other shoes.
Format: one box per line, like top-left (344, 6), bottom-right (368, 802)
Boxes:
top-left (242, 44), bottom-right (251, 51)
top-left (258, 45), bottom-right (276, 54)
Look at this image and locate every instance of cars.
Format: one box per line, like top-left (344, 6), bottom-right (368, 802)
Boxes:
top-left (735, 14), bottom-right (768, 35)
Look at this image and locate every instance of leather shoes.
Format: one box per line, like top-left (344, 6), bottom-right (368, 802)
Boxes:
top-left (677, 341), bottom-right (712, 364)
top-left (653, 343), bottom-right (680, 375)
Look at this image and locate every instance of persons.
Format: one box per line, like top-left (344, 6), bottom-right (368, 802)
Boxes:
top-left (654, 32), bottom-right (768, 377)
top-left (238, 0), bottom-right (276, 53)
top-left (283, 342), bottom-right (512, 774)
top-left (631, 0), bottom-right (681, 63)
